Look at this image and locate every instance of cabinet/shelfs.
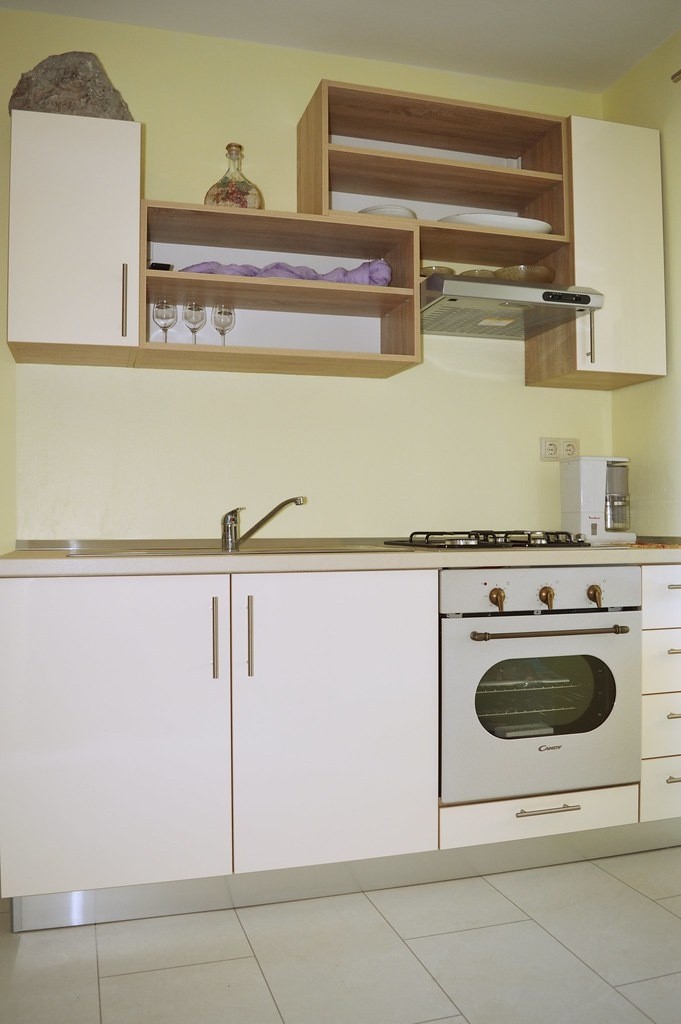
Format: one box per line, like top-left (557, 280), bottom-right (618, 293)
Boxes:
top-left (522, 115), bottom-right (668, 391)
top-left (6, 108), bottom-right (142, 369)
top-left (296, 77), bottom-right (571, 268)
top-left (439, 782), bottom-right (642, 851)
top-left (0, 569), bottom-right (439, 900)
top-left (639, 562), bottom-right (681, 823)
top-left (133, 197), bottom-right (422, 379)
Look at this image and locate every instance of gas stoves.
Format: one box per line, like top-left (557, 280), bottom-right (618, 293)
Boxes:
top-left (384, 530), bottom-right (591, 548)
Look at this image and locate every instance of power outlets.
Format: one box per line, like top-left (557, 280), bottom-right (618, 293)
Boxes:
top-left (560, 437), bottom-right (580, 462)
top-left (540, 438), bottom-right (560, 462)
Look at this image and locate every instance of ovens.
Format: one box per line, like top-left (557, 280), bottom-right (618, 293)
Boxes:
top-left (440, 565), bottom-right (642, 852)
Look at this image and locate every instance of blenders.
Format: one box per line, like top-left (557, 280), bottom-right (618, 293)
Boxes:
top-left (560, 456), bottom-right (636, 548)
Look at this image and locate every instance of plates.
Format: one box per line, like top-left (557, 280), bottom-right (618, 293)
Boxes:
top-left (437, 213), bottom-right (552, 234)
top-left (358, 204), bottom-right (418, 219)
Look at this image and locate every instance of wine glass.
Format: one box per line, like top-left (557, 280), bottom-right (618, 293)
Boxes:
top-left (153, 295), bottom-right (178, 343)
top-left (182, 302), bottom-right (207, 344)
top-left (211, 302), bottom-right (236, 346)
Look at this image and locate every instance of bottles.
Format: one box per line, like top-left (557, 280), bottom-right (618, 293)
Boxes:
top-left (204, 142), bottom-right (265, 211)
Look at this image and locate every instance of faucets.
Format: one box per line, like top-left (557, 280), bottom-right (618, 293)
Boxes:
top-left (221, 495), bottom-right (308, 552)
